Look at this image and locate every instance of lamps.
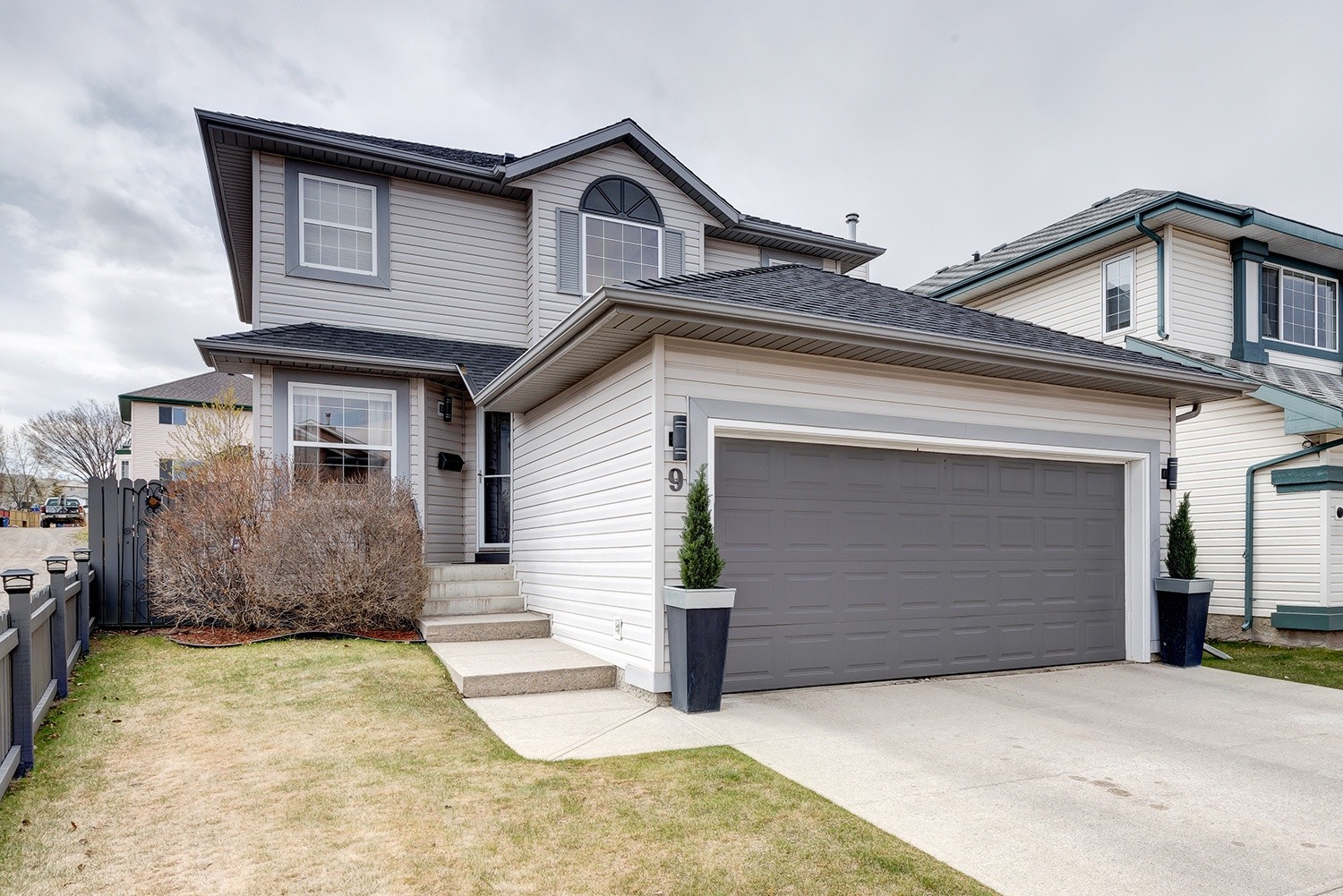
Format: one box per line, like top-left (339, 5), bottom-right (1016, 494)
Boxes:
top-left (1162, 457), bottom-right (1178, 489)
top-left (42, 556), bottom-right (71, 573)
top-left (440, 396), bottom-right (452, 422)
top-left (70, 548), bottom-right (94, 562)
top-left (669, 415), bottom-right (687, 461)
top-left (0, 569), bottom-right (38, 595)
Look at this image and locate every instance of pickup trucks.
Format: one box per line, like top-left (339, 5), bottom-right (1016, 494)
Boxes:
top-left (39, 498), bottom-right (84, 528)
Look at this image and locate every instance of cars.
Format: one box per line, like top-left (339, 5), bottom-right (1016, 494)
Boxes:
top-left (83, 504), bottom-right (89, 514)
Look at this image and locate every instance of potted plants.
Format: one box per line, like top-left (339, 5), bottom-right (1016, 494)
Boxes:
top-left (1155, 491), bottom-right (1214, 670)
top-left (664, 460), bottom-right (737, 714)
